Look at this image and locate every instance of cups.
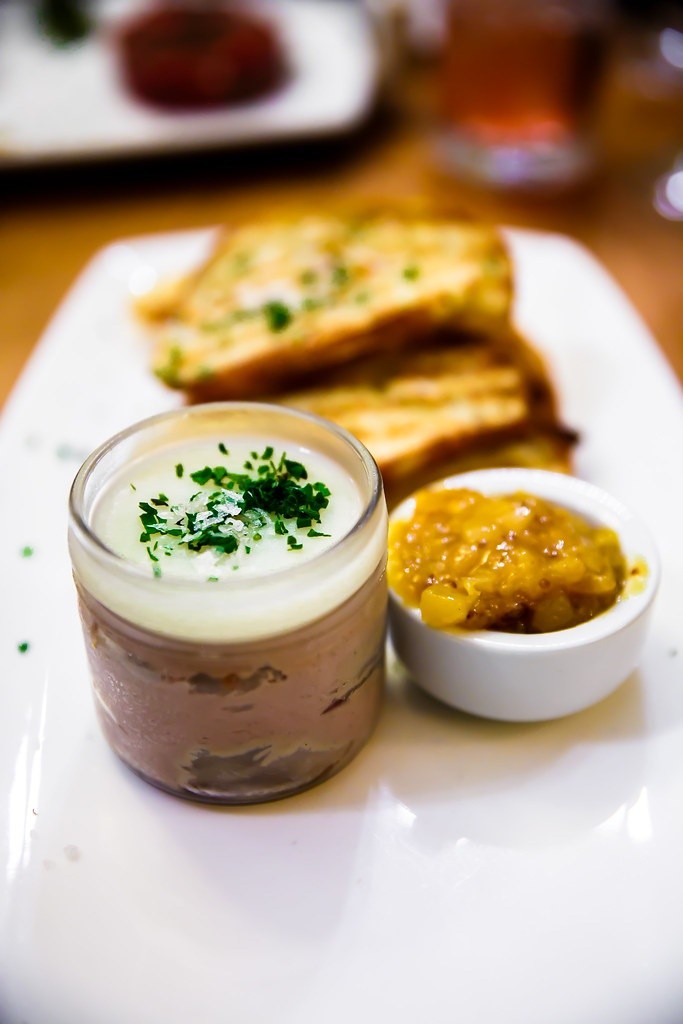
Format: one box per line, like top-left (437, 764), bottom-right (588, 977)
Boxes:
top-left (67, 402), bottom-right (387, 804)
top-left (438, 0), bottom-right (605, 201)
top-left (389, 467), bottom-right (660, 721)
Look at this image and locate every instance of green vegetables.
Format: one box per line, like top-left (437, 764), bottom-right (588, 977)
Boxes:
top-left (129, 441), bottom-right (332, 582)
top-left (152, 250), bottom-right (428, 380)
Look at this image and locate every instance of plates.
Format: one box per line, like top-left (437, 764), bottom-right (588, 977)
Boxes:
top-left (0, 225), bottom-right (680, 1024)
top-left (0, 0), bottom-right (385, 169)
